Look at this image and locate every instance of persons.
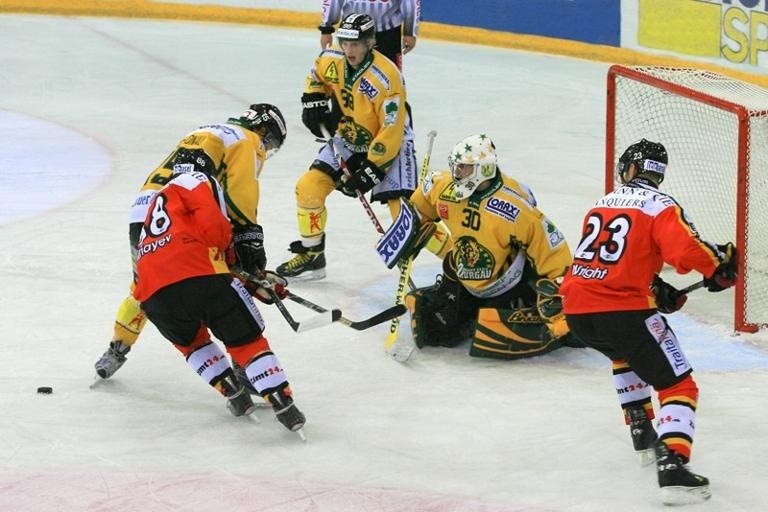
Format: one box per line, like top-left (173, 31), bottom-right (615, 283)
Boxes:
top-left (130, 169), bottom-right (315, 446)
top-left (86, 102), bottom-right (288, 394)
top-left (375, 134), bottom-right (591, 362)
top-left (277, 10), bottom-right (454, 286)
top-left (559, 136), bottom-right (740, 507)
top-left (318, 0), bottom-right (422, 138)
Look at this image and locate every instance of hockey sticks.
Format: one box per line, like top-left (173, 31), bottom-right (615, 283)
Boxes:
top-left (385, 130), bottom-right (438, 363)
top-left (253, 263), bottom-right (342, 335)
top-left (241, 271), bottom-right (408, 331)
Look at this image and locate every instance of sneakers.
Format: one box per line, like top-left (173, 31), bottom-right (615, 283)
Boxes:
top-left (276, 233), bottom-right (326, 278)
top-left (652, 440), bottom-right (711, 490)
top-left (94, 340), bottom-right (132, 379)
top-left (225, 383), bottom-right (254, 417)
top-left (628, 422), bottom-right (658, 452)
top-left (267, 388), bottom-right (307, 432)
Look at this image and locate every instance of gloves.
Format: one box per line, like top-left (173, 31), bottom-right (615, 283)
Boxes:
top-left (300, 92), bottom-right (330, 138)
top-left (651, 280), bottom-right (688, 314)
top-left (342, 158), bottom-right (386, 201)
top-left (232, 224), bottom-right (267, 275)
top-left (702, 262), bottom-right (735, 293)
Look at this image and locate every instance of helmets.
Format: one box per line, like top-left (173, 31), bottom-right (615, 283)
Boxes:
top-left (167, 148), bottom-right (213, 175)
top-left (615, 139), bottom-right (669, 184)
top-left (238, 103), bottom-right (288, 160)
top-left (335, 12), bottom-right (376, 51)
top-left (448, 132), bottom-right (498, 202)
